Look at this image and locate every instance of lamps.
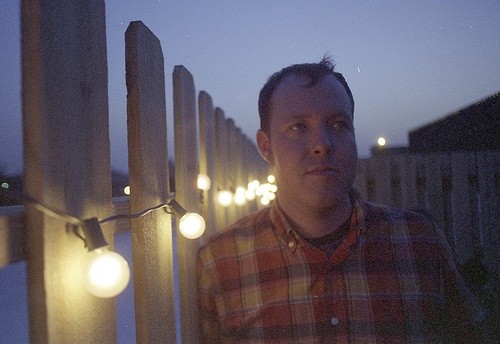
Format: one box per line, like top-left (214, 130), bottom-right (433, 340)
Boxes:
top-left (74, 217), bottom-right (130, 299)
top-left (163, 199), bottom-right (206, 239)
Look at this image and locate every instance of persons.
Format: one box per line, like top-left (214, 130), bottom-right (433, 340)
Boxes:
top-left (197, 52), bottom-right (500, 344)
